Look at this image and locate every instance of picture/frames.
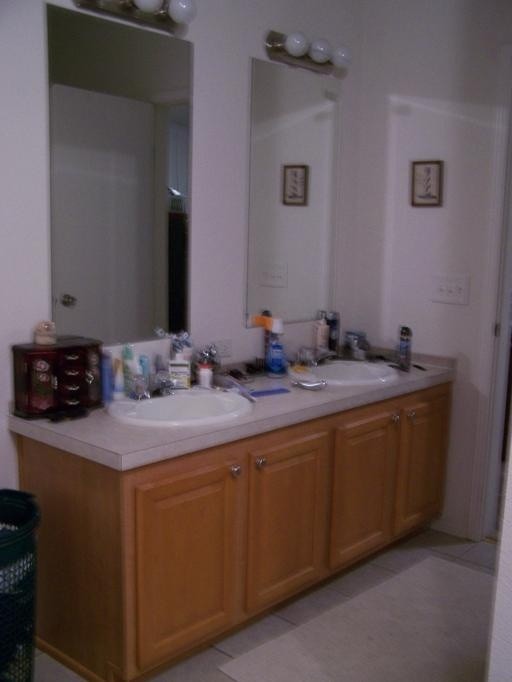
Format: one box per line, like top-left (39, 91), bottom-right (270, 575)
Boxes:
top-left (408, 159), bottom-right (444, 209)
top-left (280, 162), bottom-right (308, 208)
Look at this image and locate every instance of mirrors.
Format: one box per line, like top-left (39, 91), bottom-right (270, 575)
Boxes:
top-left (240, 54), bottom-right (345, 331)
top-left (37, 2), bottom-right (193, 350)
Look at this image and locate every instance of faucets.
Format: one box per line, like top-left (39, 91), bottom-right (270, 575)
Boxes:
top-left (158, 379), bottom-right (176, 396)
top-left (303, 349), bottom-right (338, 368)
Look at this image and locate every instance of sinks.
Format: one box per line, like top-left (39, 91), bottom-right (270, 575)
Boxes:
top-left (106, 385), bottom-right (252, 428)
top-left (288, 360), bottom-right (398, 386)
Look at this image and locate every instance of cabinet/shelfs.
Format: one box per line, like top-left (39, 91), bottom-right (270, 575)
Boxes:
top-left (14, 401), bottom-right (331, 682)
top-left (325, 371), bottom-right (455, 582)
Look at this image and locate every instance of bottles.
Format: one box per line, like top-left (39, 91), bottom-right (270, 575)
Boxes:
top-left (103, 343), bottom-right (150, 402)
top-left (397, 325), bottom-right (413, 369)
top-left (271, 342), bottom-right (284, 376)
top-left (315, 309), bottom-right (340, 355)
top-left (198, 358), bottom-right (213, 389)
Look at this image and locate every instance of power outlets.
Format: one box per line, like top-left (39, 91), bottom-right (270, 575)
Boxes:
top-left (427, 268), bottom-right (471, 309)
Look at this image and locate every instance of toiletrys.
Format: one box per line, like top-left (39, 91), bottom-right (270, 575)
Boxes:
top-left (268, 317), bottom-right (286, 377)
top-left (314, 309), bottom-right (414, 370)
top-left (98, 330), bottom-right (212, 404)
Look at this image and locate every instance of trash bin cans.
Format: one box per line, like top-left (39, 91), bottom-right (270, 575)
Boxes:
top-left (1, 488), bottom-right (42, 681)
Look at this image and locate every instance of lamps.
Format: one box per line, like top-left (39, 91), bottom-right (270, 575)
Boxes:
top-left (73, 0), bottom-right (198, 36)
top-left (265, 27), bottom-right (355, 79)
